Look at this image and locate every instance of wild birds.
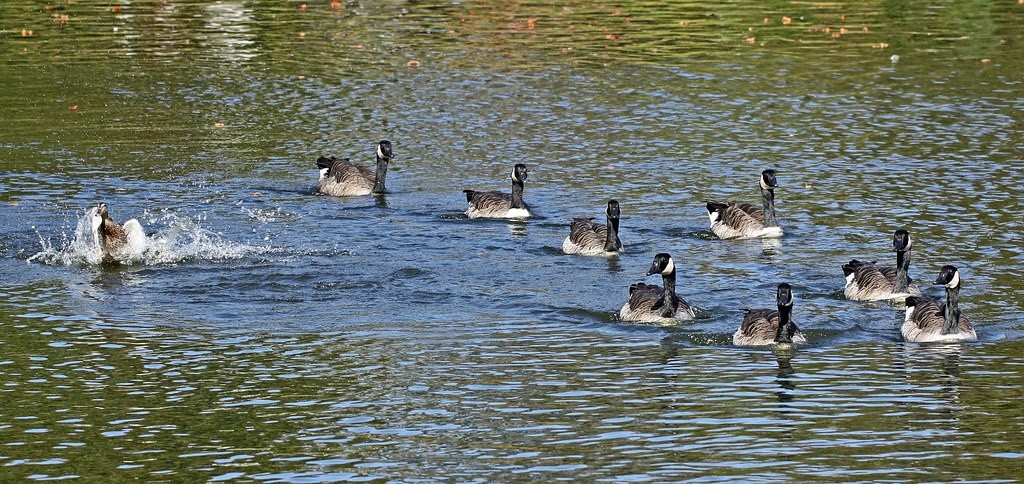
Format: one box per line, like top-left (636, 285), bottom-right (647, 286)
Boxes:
top-left (620, 252), bottom-right (696, 323)
top-left (840, 229), bottom-right (923, 302)
top-left (705, 168), bottom-right (784, 239)
top-left (563, 199), bottom-right (625, 256)
top-left (462, 163), bottom-right (530, 218)
top-left (93, 202), bottom-right (128, 265)
top-left (732, 282), bottom-right (806, 346)
top-left (901, 265), bottom-right (978, 343)
top-left (318, 139), bottom-right (394, 196)
